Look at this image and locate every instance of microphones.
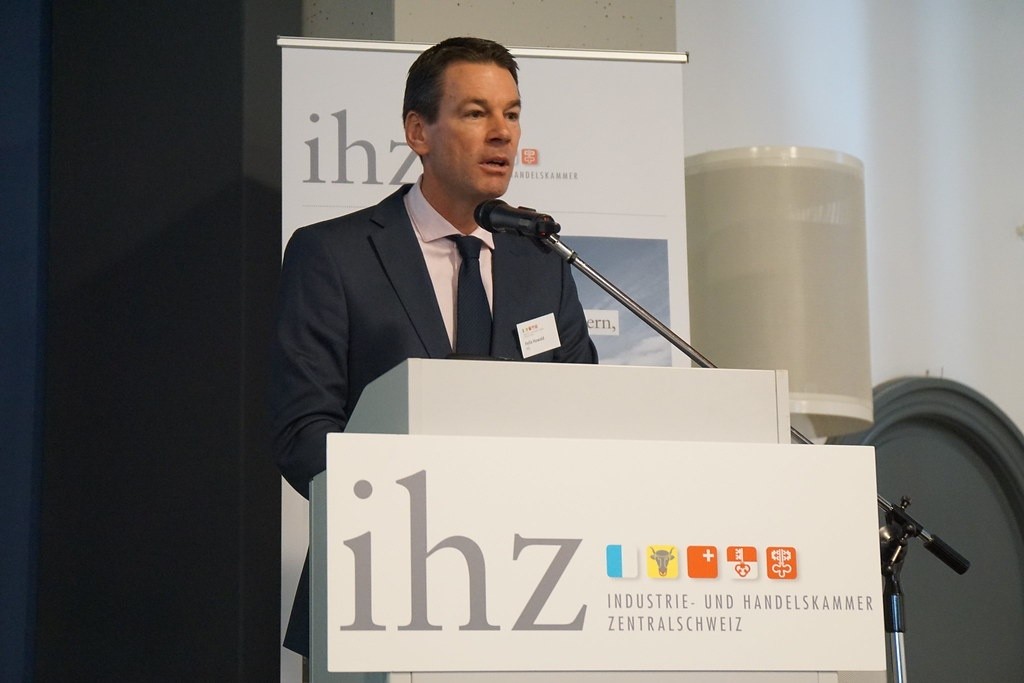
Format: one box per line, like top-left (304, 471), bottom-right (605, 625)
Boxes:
top-left (474, 198), bottom-right (561, 238)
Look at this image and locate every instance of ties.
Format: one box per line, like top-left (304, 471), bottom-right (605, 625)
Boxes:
top-left (444, 234), bottom-right (492, 359)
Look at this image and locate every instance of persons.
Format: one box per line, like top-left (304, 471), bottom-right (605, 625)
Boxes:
top-left (264, 37), bottom-right (598, 683)
